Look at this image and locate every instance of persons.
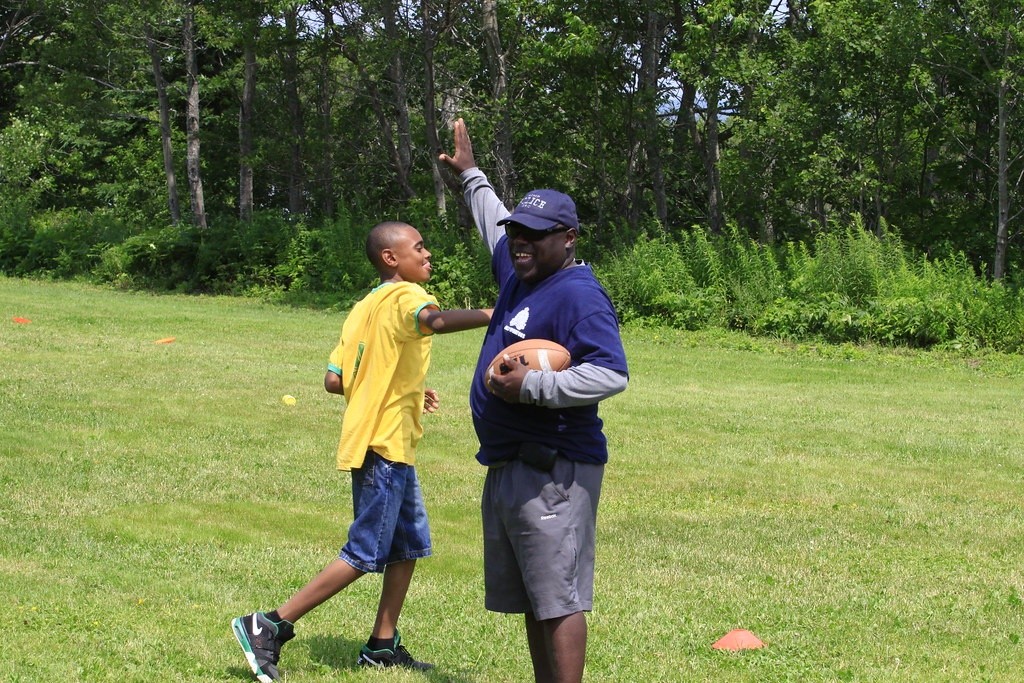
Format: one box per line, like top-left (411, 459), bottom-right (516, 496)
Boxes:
top-left (227, 221), bottom-right (500, 682)
top-left (438, 117), bottom-right (632, 683)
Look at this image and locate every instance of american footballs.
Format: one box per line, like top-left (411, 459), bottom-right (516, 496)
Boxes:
top-left (483, 338), bottom-right (572, 403)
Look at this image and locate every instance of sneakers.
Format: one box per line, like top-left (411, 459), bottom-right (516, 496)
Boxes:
top-left (231, 612), bottom-right (296, 683)
top-left (357, 628), bottom-right (434, 672)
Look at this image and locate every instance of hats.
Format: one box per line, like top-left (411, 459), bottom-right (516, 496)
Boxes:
top-left (496, 188), bottom-right (580, 234)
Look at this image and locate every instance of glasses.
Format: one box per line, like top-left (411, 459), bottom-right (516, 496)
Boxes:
top-left (505, 222), bottom-right (570, 244)
